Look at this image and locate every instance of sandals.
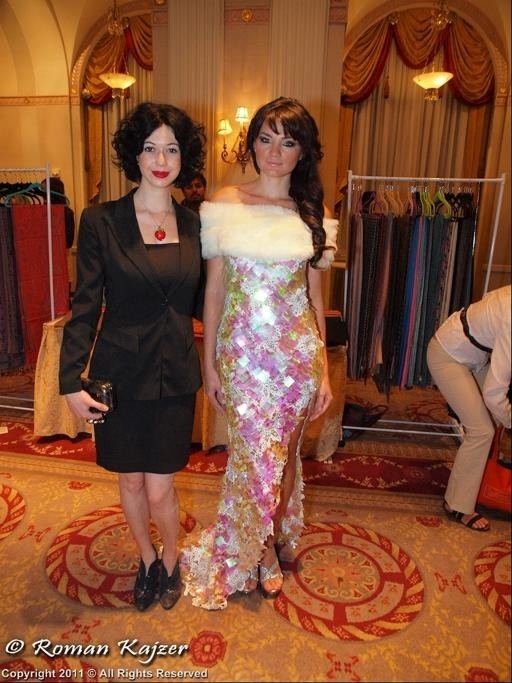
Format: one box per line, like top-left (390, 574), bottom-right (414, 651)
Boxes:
top-left (237, 562), bottom-right (259, 594)
top-left (259, 557), bottom-right (284, 596)
top-left (443, 497), bottom-right (491, 532)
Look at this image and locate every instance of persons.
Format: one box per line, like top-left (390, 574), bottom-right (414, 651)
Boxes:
top-left (425, 284), bottom-right (511, 531)
top-left (180, 95), bottom-right (343, 613)
top-left (177, 170), bottom-right (211, 212)
top-left (57, 100), bottom-right (208, 611)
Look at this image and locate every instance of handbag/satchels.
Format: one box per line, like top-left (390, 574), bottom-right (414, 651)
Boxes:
top-left (80, 376), bottom-right (115, 424)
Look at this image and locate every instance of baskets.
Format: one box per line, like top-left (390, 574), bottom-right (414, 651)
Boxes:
top-left (342, 400), bottom-right (389, 442)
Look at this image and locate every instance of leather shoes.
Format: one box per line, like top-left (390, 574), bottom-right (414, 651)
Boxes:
top-left (133, 544), bottom-right (160, 612)
top-left (158, 548), bottom-right (182, 610)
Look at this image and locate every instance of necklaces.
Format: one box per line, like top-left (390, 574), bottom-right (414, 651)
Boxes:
top-left (135, 191), bottom-right (173, 241)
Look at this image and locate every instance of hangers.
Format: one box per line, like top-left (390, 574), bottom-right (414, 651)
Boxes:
top-left (1, 167), bottom-right (73, 208)
top-left (352, 176), bottom-right (481, 220)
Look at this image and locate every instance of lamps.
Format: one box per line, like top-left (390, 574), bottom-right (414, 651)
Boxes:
top-left (97, 1), bottom-right (138, 98)
top-left (413, 1), bottom-right (453, 102)
